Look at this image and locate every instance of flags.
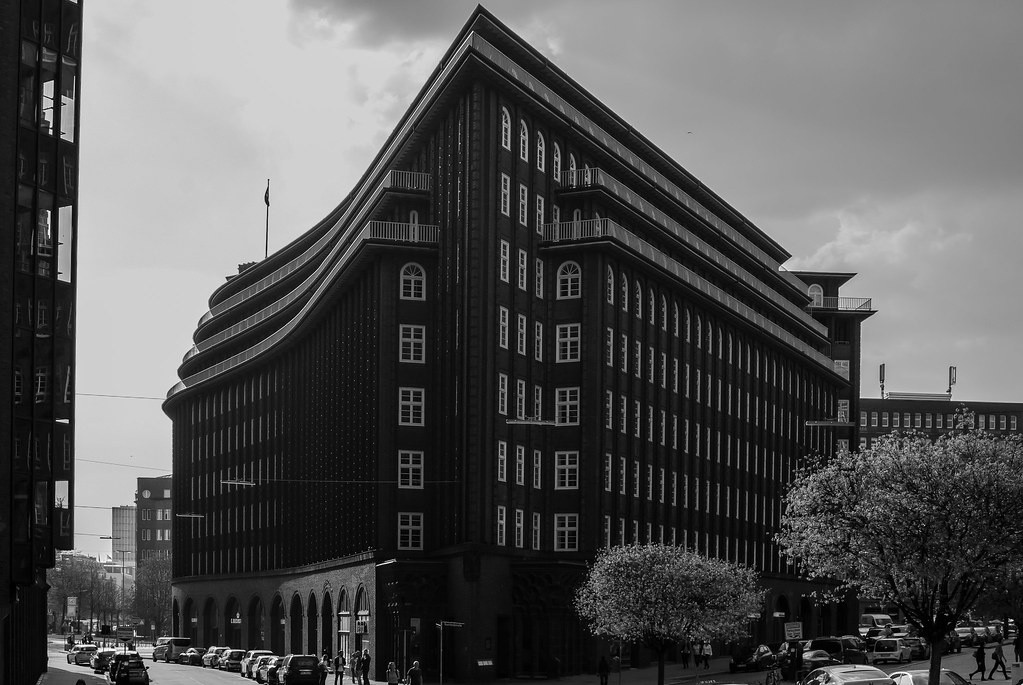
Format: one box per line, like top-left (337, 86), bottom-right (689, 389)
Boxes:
top-left (264, 186), bottom-right (270, 206)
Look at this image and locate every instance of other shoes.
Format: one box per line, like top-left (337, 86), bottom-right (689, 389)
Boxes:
top-left (988, 677), bottom-right (995, 680)
top-left (980, 677), bottom-right (987, 680)
top-left (1006, 676), bottom-right (1011, 680)
top-left (969, 673), bottom-right (972, 680)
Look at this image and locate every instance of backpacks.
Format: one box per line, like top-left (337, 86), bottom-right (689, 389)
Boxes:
top-left (68, 635), bottom-right (71, 643)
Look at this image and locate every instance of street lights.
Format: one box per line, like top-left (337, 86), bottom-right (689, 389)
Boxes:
top-left (116, 550), bottom-right (132, 626)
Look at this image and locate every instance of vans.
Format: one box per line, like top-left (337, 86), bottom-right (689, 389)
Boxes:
top-left (152, 637), bottom-right (192, 663)
top-left (811, 638), bottom-right (869, 665)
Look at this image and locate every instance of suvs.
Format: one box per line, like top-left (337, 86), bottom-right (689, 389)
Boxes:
top-left (275, 653), bottom-right (320, 685)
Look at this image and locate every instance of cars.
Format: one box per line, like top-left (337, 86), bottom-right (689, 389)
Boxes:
top-left (219, 650), bottom-right (247, 672)
top-left (777, 635), bottom-right (868, 661)
top-left (729, 644), bottom-right (776, 673)
top-left (90, 649), bottom-right (116, 670)
top-left (781, 650), bottom-right (842, 681)
top-left (178, 648), bottom-right (208, 666)
top-left (256, 657), bottom-right (285, 685)
top-left (108, 653), bottom-right (144, 682)
top-left (201, 646), bottom-right (230, 669)
top-left (114, 659), bottom-right (149, 685)
top-left (239, 650), bottom-right (273, 679)
top-left (800, 664), bottom-right (897, 684)
top-left (67, 644), bottom-right (97, 666)
top-left (251, 656), bottom-right (279, 681)
top-left (860, 613), bottom-right (1017, 665)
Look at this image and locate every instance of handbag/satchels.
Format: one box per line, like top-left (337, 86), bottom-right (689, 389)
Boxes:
top-left (992, 652), bottom-right (998, 660)
top-left (972, 651), bottom-right (978, 658)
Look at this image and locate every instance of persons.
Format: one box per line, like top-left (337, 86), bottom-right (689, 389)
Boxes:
top-left (969, 641), bottom-right (988, 681)
top-left (1013, 633), bottom-right (1023, 662)
top-left (988, 637), bottom-right (1012, 680)
top-left (70, 633), bottom-right (76, 649)
top-left (387, 662), bottom-right (400, 685)
top-left (82, 632), bottom-right (91, 644)
top-left (407, 661), bottom-right (423, 685)
top-left (317, 649), bottom-right (371, 685)
top-left (597, 654), bottom-right (610, 685)
top-left (680, 638), bottom-right (712, 672)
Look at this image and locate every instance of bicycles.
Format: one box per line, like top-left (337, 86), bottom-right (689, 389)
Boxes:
top-left (765, 663), bottom-right (781, 685)
